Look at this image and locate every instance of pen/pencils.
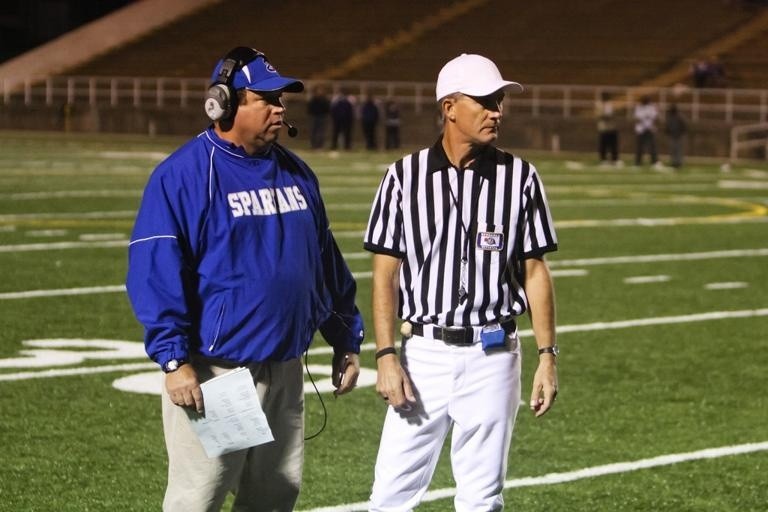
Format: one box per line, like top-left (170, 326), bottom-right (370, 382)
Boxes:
top-left (336, 355), bottom-right (349, 399)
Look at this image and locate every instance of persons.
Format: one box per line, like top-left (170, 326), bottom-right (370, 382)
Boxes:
top-left (632, 95), bottom-right (661, 170)
top-left (665, 105), bottom-right (684, 169)
top-left (305, 86), bottom-right (401, 151)
top-left (123, 45), bottom-right (362, 512)
top-left (595, 92), bottom-right (622, 168)
top-left (363, 53), bottom-right (559, 512)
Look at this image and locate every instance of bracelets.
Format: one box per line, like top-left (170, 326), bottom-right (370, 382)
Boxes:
top-left (375, 347), bottom-right (397, 362)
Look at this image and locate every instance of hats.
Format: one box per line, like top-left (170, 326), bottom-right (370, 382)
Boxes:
top-left (436, 54), bottom-right (524, 103)
top-left (212, 53), bottom-right (304, 99)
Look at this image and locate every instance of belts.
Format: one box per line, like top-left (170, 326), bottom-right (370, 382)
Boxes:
top-left (405, 320), bottom-right (516, 347)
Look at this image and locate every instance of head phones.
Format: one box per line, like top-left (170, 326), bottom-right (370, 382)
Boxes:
top-left (204, 47), bottom-right (265, 119)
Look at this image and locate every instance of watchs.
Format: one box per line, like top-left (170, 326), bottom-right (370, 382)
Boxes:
top-left (537, 344), bottom-right (559, 356)
top-left (163, 358), bottom-right (187, 375)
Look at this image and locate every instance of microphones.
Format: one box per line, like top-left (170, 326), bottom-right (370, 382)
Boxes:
top-left (282, 120), bottom-right (299, 137)
